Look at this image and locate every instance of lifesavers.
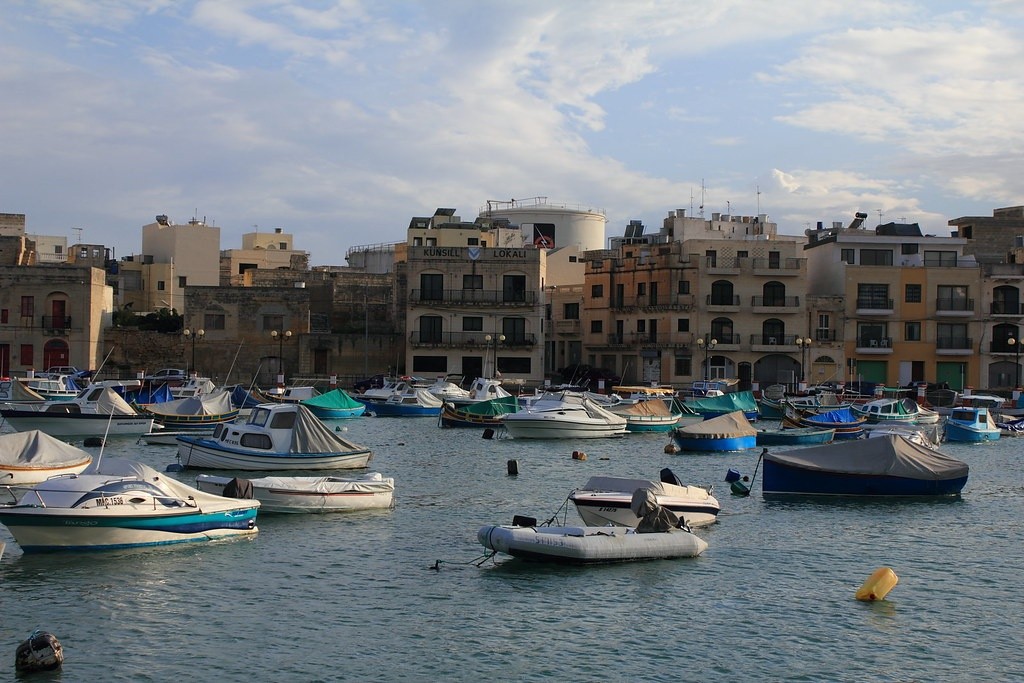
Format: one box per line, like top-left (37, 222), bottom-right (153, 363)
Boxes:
top-left (401, 376), bottom-right (411, 381)
top-left (0, 377), bottom-right (10, 381)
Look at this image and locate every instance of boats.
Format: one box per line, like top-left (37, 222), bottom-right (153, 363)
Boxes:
top-left (491, 361), bottom-right (633, 439)
top-left (365, 352), bottom-right (515, 404)
top-left (298, 388), bottom-right (367, 420)
top-left (253, 387), bottom-right (305, 405)
top-left (0, 337), bottom-right (262, 436)
top-left (438, 393), bottom-right (523, 429)
top-left (354, 387), bottom-right (455, 419)
top-left (603, 398), bottom-right (683, 433)
top-left (175, 387), bottom-right (372, 471)
top-left (195, 468), bottom-right (397, 516)
top-left (761, 431), bottom-right (970, 497)
top-left (0, 401), bottom-right (261, 553)
top-left (569, 467), bottom-right (722, 532)
top-left (666, 409), bottom-right (760, 452)
top-left (516, 360), bottom-right (1024, 451)
top-left (0, 428), bottom-right (96, 485)
top-left (476, 490), bottom-right (710, 564)
top-left (756, 426), bottom-right (837, 448)
top-left (263, 385), bottom-right (322, 404)
top-left (943, 406), bottom-right (1002, 443)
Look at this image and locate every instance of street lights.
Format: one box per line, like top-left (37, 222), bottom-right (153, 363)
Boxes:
top-left (269, 325), bottom-right (293, 389)
top-left (484, 334), bottom-right (506, 378)
top-left (183, 327), bottom-right (204, 376)
top-left (697, 333), bottom-right (720, 382)
top-left (1008, 338), bottom-right (1024, 390)
top-left (795, 337), bottom-right (813, 391)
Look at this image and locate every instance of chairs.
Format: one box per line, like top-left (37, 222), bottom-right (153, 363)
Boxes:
top-left (870, 340), bottom-right (878, 347)
top-left (880, 340), bottom-right (888, 347)
top-left (769, 337), bottom-right (776, 345)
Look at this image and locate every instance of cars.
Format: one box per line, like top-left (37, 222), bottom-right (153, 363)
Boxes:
top-left (559, 363), bottom-right (591, 382)
top-left (35, 366), bottom-right (80, 379)
top-left (143, 368), bottom-right (188, 380)
top-left (353, 374), bottom-right (389, 392)
top-left (581, 368), bottom-right (622, 390)
